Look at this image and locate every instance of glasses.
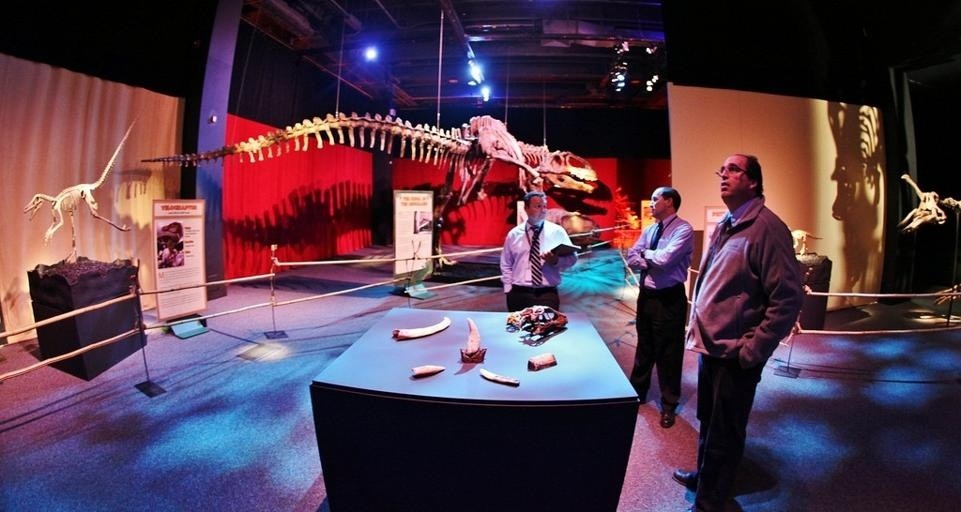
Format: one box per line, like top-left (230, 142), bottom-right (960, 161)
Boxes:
top-left (715, 166), bottom-right (746, 178)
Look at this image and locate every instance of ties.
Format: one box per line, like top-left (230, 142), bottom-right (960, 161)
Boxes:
top-left (530, 224), bottom-right (542, 286)
top-left (640, 220), bottom-right (663, 288)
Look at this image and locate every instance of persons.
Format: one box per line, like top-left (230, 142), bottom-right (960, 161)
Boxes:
top-left (671, 153), bottom-right (803, 511)
top-left (500, 191), bottom-right (578, 313)
top-left (627, 187), bottom-right (696, 428)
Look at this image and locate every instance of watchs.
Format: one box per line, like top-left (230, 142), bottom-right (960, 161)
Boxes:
top-left (640, 247), bottom-right (648, 259)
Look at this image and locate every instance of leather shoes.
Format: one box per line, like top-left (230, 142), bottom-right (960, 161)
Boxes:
top-left (672, 468), bottom-right (697, 486)
top-left (638, 396), bottom-right (646, 404)
top-left (660, 410), bottom-right (675, 428)
